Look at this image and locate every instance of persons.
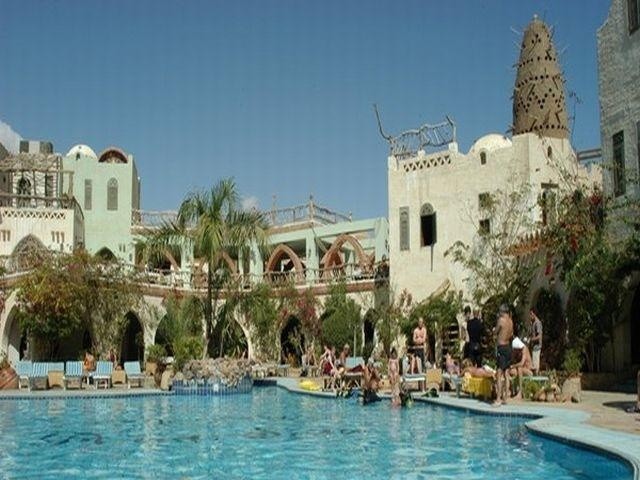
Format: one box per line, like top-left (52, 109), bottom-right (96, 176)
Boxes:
top-left (81, 347), bottom-right (122, 385)
top-left (300, 304), bottom-right (543, 408)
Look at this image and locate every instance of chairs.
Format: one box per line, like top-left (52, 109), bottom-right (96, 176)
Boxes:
top-left (252, 355), bottom-right (547, 402)
top-left (18, 361), bottom-right (146, 389)
